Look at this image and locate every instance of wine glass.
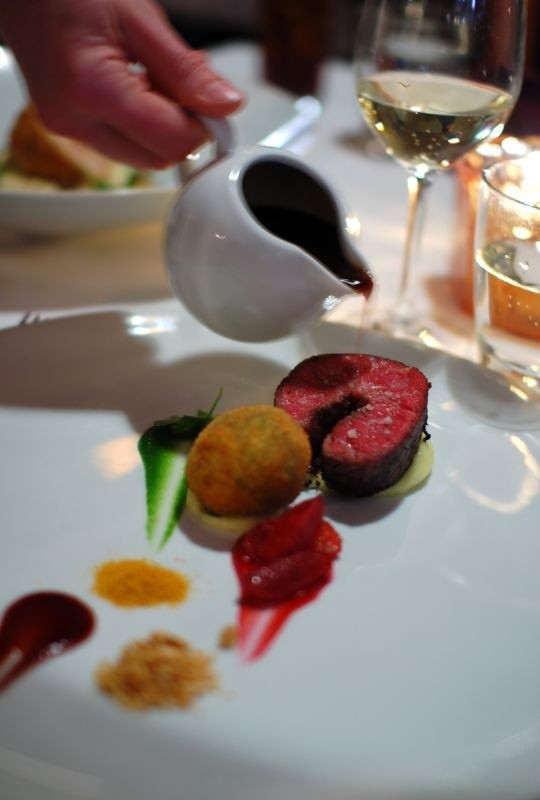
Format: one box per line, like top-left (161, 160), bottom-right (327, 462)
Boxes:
top-left (319, 0), bottom-right (526, 347)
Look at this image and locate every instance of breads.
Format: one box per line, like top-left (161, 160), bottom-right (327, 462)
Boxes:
top-left (8, 98), bottom-right (137, 191)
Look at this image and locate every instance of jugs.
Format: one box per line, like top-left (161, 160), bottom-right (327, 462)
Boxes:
top-left (164, 114), bottom-right (374, 343)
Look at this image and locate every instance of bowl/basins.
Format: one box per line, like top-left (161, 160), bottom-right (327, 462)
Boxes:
top-left (0, 62), bottom-right (233, 224)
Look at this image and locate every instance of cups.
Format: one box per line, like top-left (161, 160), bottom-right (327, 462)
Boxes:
top-left (481, 154), bottom-right (540, 380)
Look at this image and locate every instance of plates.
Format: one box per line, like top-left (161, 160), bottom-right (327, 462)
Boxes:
top-left (0, 307), bottom-right (540, 800)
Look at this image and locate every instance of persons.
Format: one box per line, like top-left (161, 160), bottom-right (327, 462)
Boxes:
top-left (0, 0), bottom-right (248, 174)
